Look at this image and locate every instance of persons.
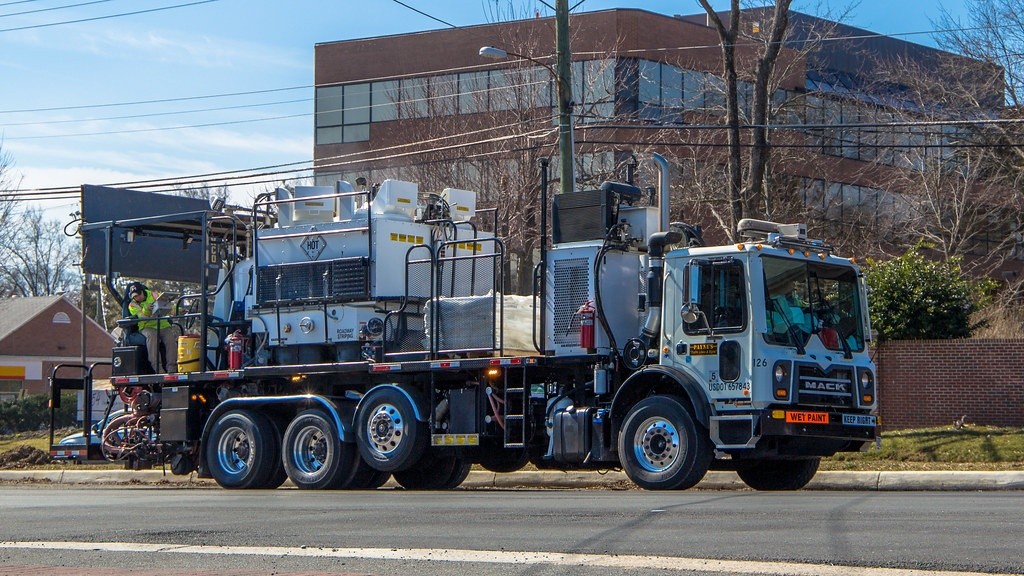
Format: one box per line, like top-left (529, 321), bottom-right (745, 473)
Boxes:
top-left (129, 283), bottom-right (178, 374)
top-left (736, 281), bottom-right (794, 328)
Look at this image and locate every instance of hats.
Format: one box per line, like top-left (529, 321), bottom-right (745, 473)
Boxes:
top-left (129, 285), bottom-right (141, 298)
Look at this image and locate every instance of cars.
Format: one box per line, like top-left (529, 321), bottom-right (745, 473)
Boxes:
top-left (56, 408), bottom-right (160, 461)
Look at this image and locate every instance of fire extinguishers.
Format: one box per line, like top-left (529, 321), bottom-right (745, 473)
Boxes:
top-left (224, 329), bottom-right (242, 370)
top-left (565, 300), bottom-right (595, 348)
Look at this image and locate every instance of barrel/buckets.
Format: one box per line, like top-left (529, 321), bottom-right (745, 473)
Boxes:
top-left (177, 334), bottom-right (202, 372)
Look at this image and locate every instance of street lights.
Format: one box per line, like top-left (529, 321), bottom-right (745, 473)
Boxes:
top-left (480, 45), bottom-right (574, 192)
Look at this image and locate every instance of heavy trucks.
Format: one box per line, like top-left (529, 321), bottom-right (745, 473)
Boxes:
top-left (64, 152), bottom-right (883, 491)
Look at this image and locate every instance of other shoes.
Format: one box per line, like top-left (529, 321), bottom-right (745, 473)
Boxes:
top-left (159, 369), bottom-right (166, 374)
top-left (167, 368), bottom-right (177, 374)
